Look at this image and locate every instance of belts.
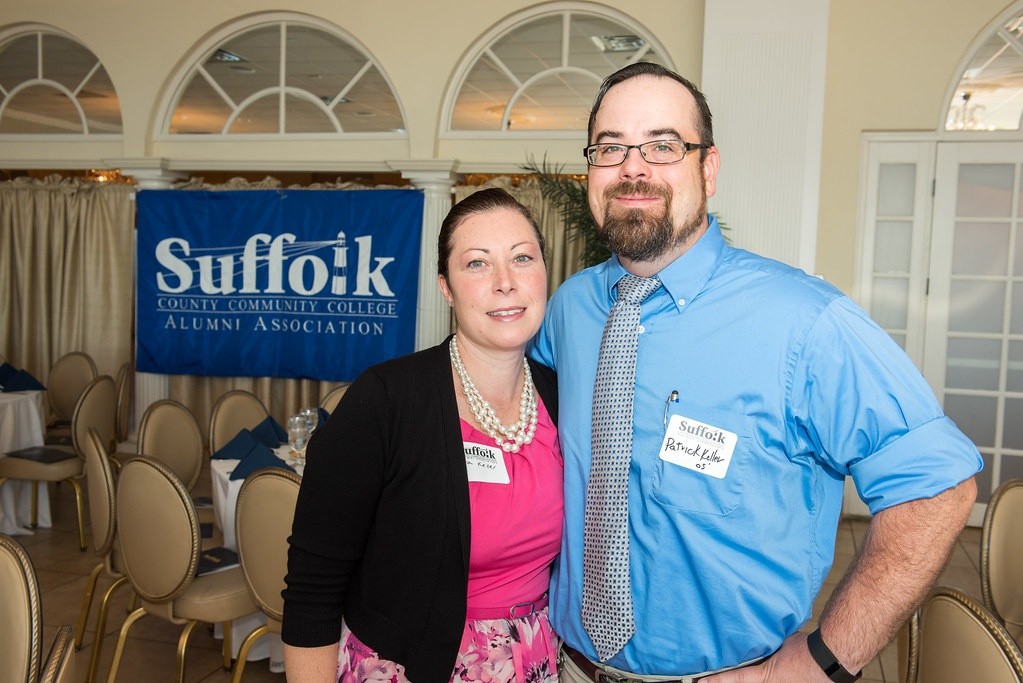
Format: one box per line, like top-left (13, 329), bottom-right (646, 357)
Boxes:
top-left (556, 635), bottom-right (775, 683)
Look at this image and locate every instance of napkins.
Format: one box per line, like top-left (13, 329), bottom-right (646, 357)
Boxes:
top-left (250, 415), bottom-right (289, 449)
top-left (229, 442), bottom-right (297, 481)
top-left (2, 369), bottom-right (49, 393)
top-left (0, 363), bottom-right (19, 385)
top-left (209, 427), bottom-right (274, 460)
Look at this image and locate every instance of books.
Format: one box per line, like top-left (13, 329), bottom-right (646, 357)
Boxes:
top-left (195, 547), bottom-right (240, 576)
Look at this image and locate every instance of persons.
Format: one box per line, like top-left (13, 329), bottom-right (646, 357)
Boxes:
top-left (526, 62), bottom-right (984, 683)
top-left (282, 187), bottom-right (564, 683)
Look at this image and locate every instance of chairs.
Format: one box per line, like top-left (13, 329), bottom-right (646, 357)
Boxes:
top-left (0, 350), bottom-right (348, 683)
top-left (916, 585), bottom-right (1023, 683)
top-left (896, 610), bottom-right (919, 683)
top-left (979, 479), bottom-right (1023, 653)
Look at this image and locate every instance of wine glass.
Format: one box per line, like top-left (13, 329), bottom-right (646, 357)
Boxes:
top-left (288, 407), bottom-right (318, 464)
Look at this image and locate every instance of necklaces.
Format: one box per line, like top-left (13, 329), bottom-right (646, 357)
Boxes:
top-left (449, 334), bottom-right (538, 453)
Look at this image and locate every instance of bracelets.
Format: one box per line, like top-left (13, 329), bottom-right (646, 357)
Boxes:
top-left (807, 628), bottom-right (862, 683)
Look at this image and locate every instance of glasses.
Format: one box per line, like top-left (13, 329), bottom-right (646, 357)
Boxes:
top-left (583, 139), bottom-right (708, 168)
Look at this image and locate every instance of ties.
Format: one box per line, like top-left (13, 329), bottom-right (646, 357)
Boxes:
top-left (580, 274), bottom-right (664, 663)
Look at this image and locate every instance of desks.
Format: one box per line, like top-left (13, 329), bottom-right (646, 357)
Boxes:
top-left (0, 390), bottom-right (70, 536)
top-left (210, 442), bottom-right (306, 674)
